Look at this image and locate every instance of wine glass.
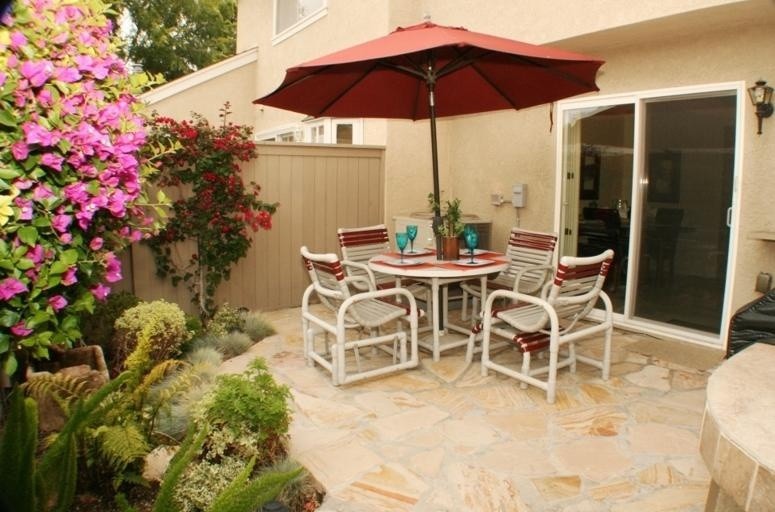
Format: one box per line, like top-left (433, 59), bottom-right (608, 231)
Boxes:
top-left (463, 223), bottom-right (479, 266)
top-left (395, 224), bottom-right (419, 265)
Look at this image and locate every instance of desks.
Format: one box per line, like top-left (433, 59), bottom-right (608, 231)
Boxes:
top-left (612, 218), bottom-right (696, 235)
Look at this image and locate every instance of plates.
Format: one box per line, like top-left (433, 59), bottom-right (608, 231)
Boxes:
top-left (454, 259), bottom-right (495, 267)
top-left (385, 256), bottom-right (425, 266)
top-left (397, 247), bottom-right (434, 258)
top-left (458, 248), bottom-right (487, 257)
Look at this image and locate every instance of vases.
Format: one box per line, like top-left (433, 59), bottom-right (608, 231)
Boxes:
top-left (27, 345), bottom-right (112, 436)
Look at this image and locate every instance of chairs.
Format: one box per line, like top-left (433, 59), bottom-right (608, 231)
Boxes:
top-left (465, 248), bottom-right (615, 403)
top-left (300, 246), bottom-right (418, 387)
top-left (583, 206), bottom-right (628, 293)
top-left (645, 206), bottom-right (685, 288)
top-left (337, 224), bottom-right (435, 355)
top-left (459, 227), bottom-right (559, 359)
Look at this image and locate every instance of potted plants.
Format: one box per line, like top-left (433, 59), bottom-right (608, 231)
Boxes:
top-left (428, 190), bottom-right (465, 261)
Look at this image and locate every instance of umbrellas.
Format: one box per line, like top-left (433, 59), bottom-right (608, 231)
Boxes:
top-left (250, 10), bottom-right (606, 261)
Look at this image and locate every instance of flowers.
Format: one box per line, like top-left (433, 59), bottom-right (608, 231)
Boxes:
top-left (0, 0), bottom-right (160, 384)
